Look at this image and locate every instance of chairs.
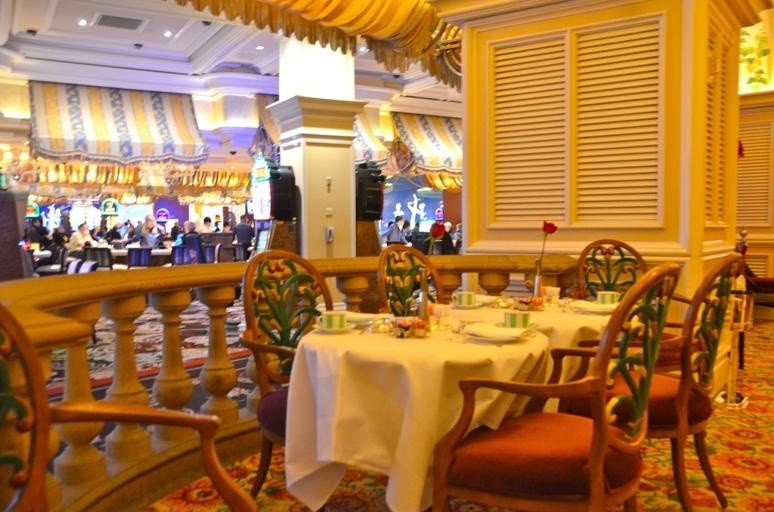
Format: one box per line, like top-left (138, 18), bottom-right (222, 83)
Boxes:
top-left (26, 241), bottom-right (223, 276)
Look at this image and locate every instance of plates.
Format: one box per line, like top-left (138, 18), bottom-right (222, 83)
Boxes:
top-left (575, 303), bottom-right (620, 313)
top-left (463, 334), bottom-right (518, 343)
top-left (311, 322), bottom-right (356, 334)
top-left (450, 302), bottom-right (482, 308)
top-left (496, 323), bottom-right (538, 329)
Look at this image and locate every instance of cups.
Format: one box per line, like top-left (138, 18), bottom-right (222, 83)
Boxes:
top-left (317, 311), bottom-right (346, 330)
top-left (597, 291), bottom-right (620, 304)
top-left (540, 286), bottom-right (562, 311)
top-left (452, 291), bottom-right (476, 306)
top-left (503, 312), bottom-right (530, 328)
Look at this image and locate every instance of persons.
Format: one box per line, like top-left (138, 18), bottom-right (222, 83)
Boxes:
top-left (377, 215), bottom-right (464, 258)
top-left (25, 212), bottom-right (254, 268)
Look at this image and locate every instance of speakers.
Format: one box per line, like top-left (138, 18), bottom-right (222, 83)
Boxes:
top-left (252, 179), bottom-right (295, 221)
top-left (356, 180), bottom-right (384, 222)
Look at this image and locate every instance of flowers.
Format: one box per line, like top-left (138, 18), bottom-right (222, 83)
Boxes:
top-left (534, 218), bottom-right (560, 276)
top-left (418, 218), bottom-right (445, 281)
